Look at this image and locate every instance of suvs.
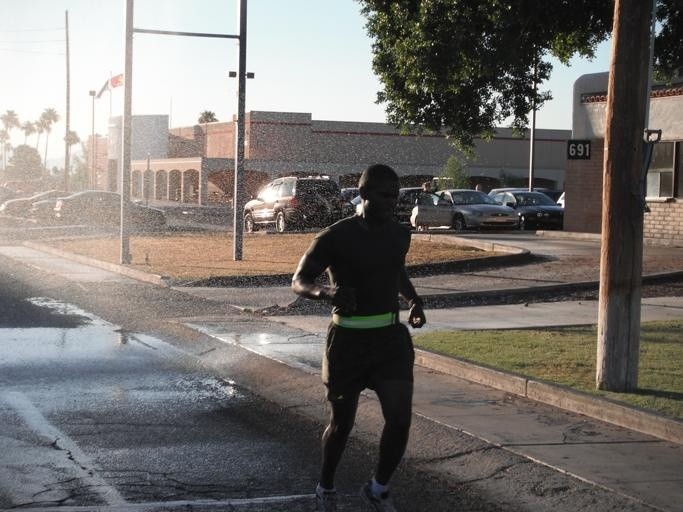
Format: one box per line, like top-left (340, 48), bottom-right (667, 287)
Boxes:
top-left (242, 173), bottom-right (342, 235)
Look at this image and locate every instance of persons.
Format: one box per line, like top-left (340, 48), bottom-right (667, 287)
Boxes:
top-left (291, 164), bottom-right (426, 512)
top-left (415, 182), bottom-right (431, 232)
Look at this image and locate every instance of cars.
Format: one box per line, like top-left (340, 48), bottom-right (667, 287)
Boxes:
top-left (0, 179), bottom-right (167, 229)
top-left (338, 184), bottom-right (566, 231)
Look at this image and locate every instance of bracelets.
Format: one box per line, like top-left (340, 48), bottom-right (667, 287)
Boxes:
top-left (408, 297), bottom-right (424, 307)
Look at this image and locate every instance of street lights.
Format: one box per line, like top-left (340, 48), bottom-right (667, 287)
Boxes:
top-left (227, 71), bottom-right (257, 261)
top-left (88, 89), bottom-right (98, 189)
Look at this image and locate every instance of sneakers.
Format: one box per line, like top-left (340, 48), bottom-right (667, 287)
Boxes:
top-left (358, 478), bottom-right (395, 511)
top-left (316, 485), bottom-right (339, 512)
